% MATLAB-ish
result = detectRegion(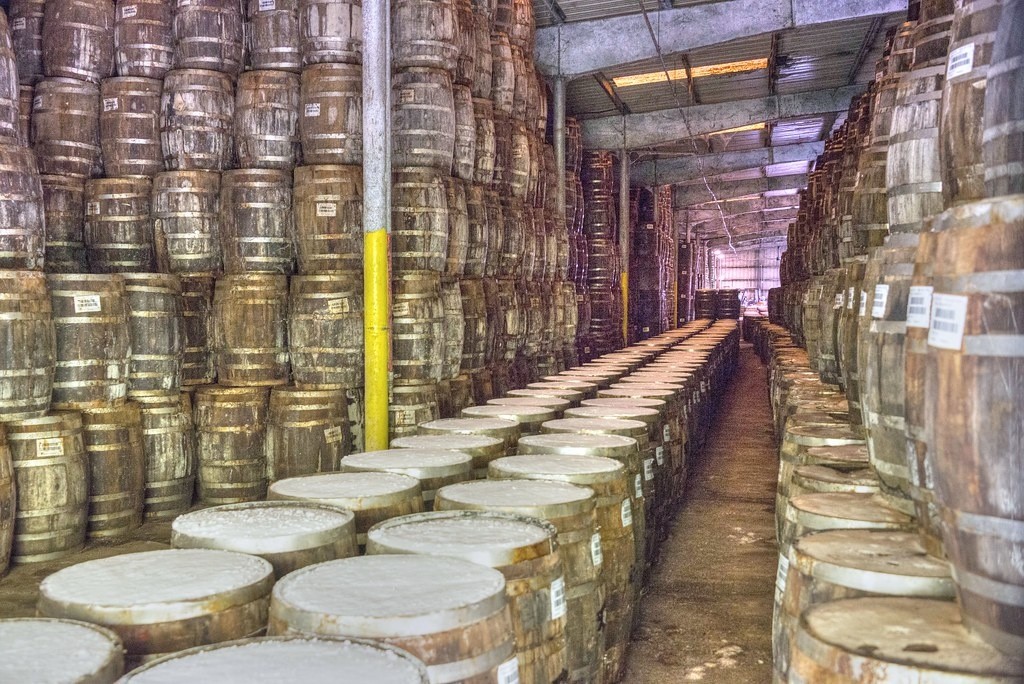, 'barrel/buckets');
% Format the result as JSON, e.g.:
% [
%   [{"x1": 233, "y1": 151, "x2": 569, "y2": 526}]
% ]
[
  {"x1": 1, "y1": 0, "x2": 740, "y2": 683},
  {"x1": 743, "y1": 0, "x2": 1024, "y2": 684}
]
